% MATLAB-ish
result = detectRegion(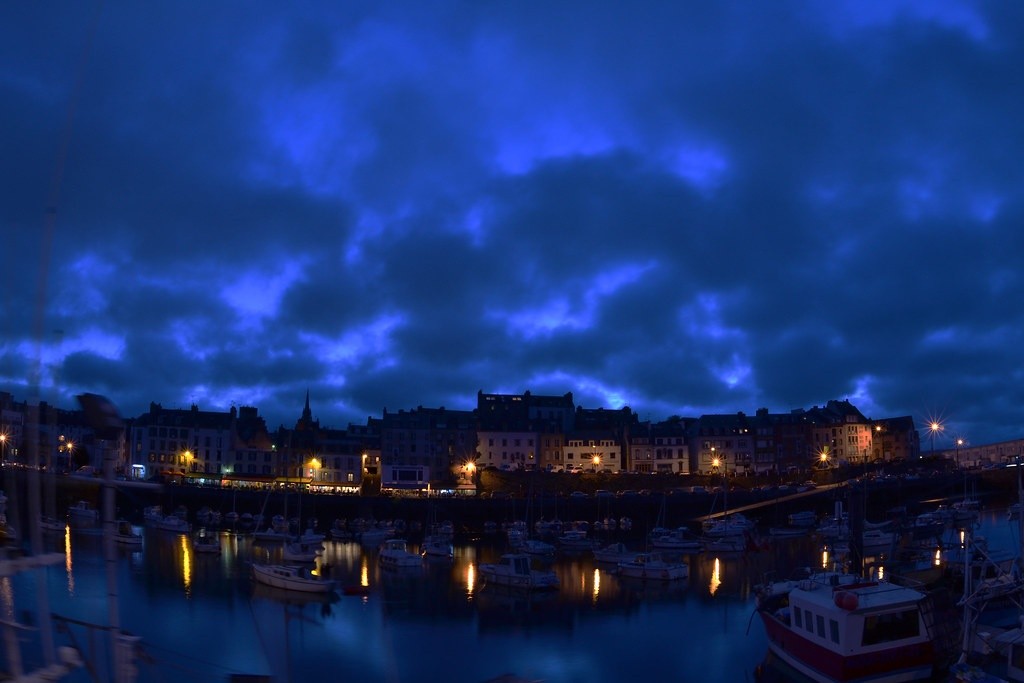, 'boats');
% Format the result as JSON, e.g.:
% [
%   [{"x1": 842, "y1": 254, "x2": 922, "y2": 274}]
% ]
[
  {"x1": 752, "y1": 552, "x2": 936, "y2": 683},
  {"x1": 0, "y1": 499, "x2": 1024, "y2": 683}
]
